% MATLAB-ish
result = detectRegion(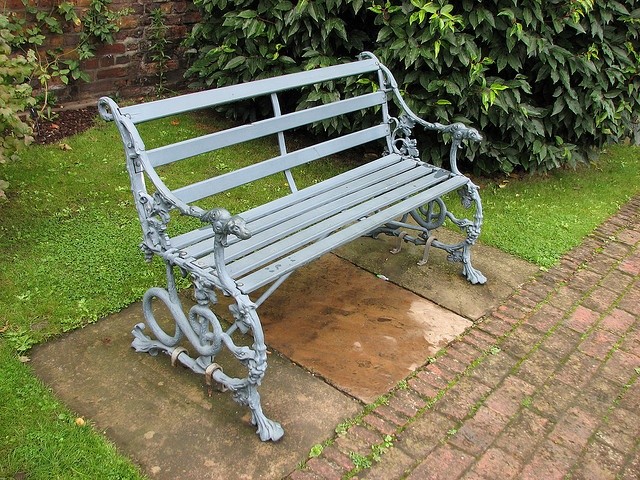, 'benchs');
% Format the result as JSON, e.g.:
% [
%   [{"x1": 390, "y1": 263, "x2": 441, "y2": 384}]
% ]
[{"x1": 98, "y1": 50, "x2": 487, "y2": 443}]
